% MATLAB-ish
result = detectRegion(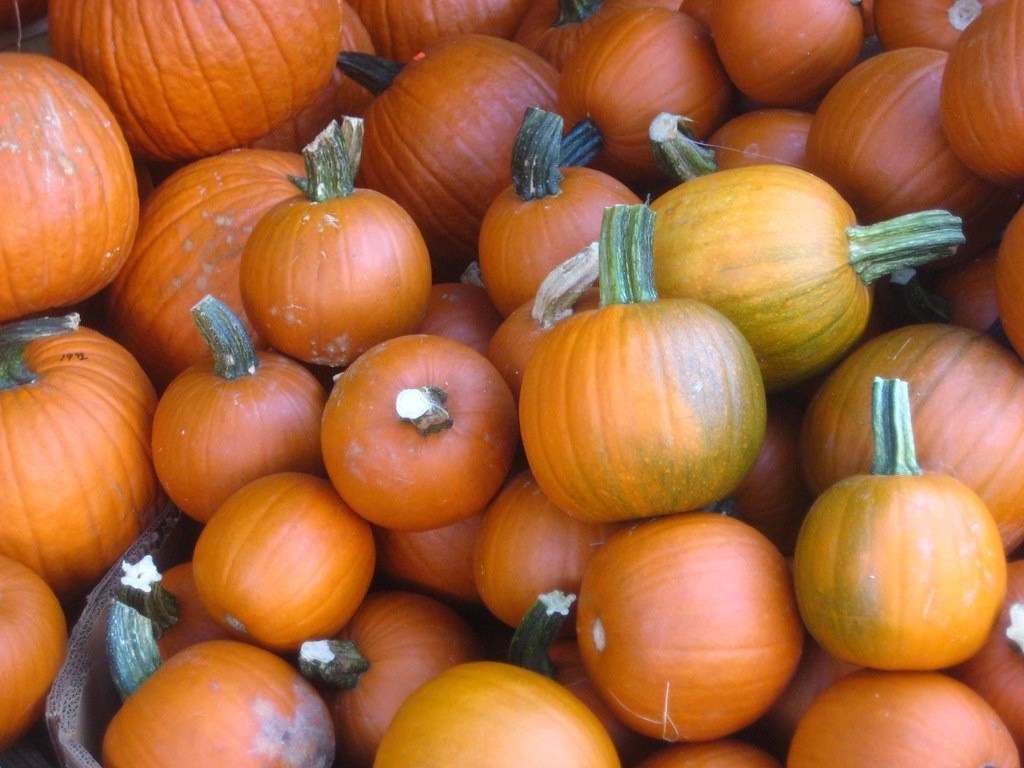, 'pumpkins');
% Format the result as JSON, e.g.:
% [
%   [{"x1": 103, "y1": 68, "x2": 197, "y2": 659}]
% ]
[{"x1": 0, "y1": 0, "x2": 1024, "y2": 768}]
